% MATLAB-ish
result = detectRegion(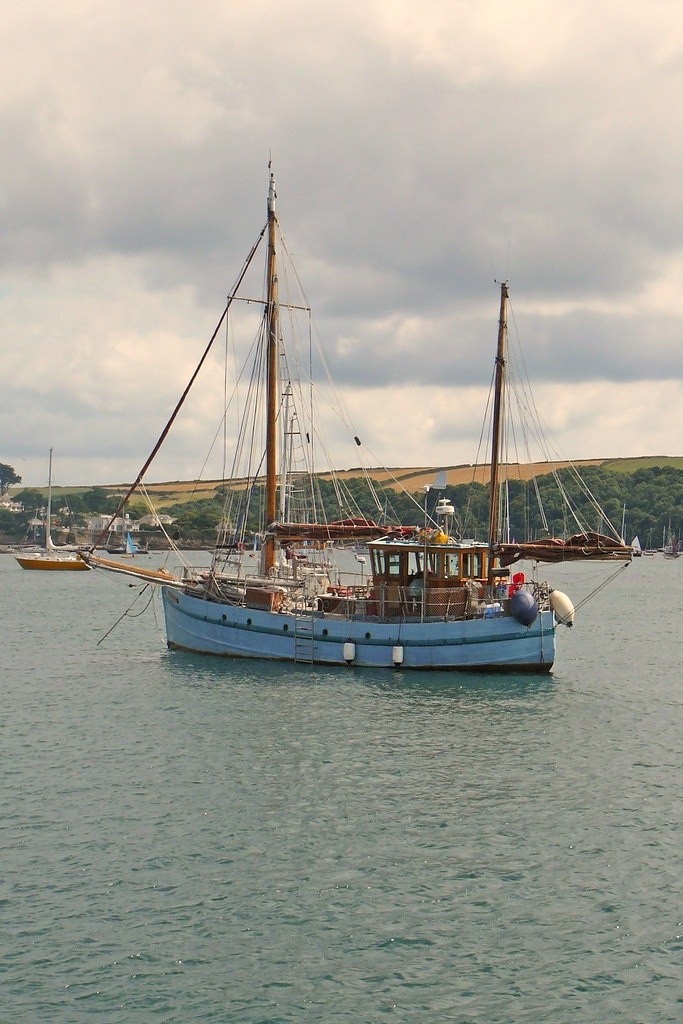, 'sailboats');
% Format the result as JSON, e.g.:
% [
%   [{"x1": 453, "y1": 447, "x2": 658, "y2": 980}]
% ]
[
  {"x1": 13, "y1": 449, "x2": 93, "y2": 571},
  {"x1": 0, "y1": 495, "x2": 683, "y2": 565},
  {"x1": 119, "y1": 530, "x2": 139, "y2": 558},
  {"x1": 65, "y1": 147, "x2": 641, "y2": 670}
]
